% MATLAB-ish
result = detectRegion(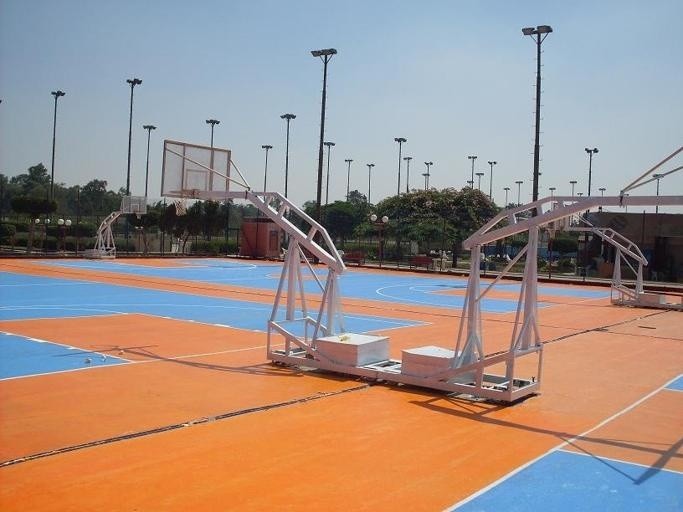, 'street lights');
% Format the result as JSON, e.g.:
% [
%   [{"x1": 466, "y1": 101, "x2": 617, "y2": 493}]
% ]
[
  {"x1": 262, "y1": 145, "x2": 272, "y2": 203},
  {"x1": 550, "y1": 181, "x2": 584, "y2": 225},
  {"x1": 404, "y1": 157, "x2": 413, "y2": 192},
  {"x1": 50, "y1": 91, "x2": 66, "y2": 201},
  {"x1": 206, "y1": 119, "x2": 220, "y2": 204},
  {"x1": 522, "y1": 25, "x2": 553, "y2": 229},
  {"x1": 599, "y1": 188, "x2": 606, "y2": 214},
  {"x1": 36, "y1": 219, "x2": 50, "y2": 248},
  {"x1": 422, "y1": 161, "x2": 433, "y2": 196},
  {"x1": 585, "y1": 147, "x2": 598, "y2": 213},
  {"x1": 653, "y1": 174, "x2": 664, "y2": 213},
  {"x1": 281, "y1": 114, "x2": 297, "y2": 200},
  {"x1": 395, "y1": 136, "x2": 407, "y2": 196},
  {"x1": 58, "y1": 219, "x2": 72, "y2": 252},
  {"x1": 344, "y1": 159, "x2": 352, "y2": 203},
  {"x1": 367, "y1": 163, "x2": 375, "y2": 204},
  {"x1": 127, "y1": 78, "x2": 143, "y2": 196},
  {"x1": 466, "y1": 155, "x2": 522, "y2": 210},
  {"x1": 371, "y1": 214, "x2": 390, "y2": 266},
  {"x1": 143, "y1": 124, "x2": 157, "y2": 196},
  {"x1": 311, "y1": 48, "x2": 338, "y2": 264}
]
